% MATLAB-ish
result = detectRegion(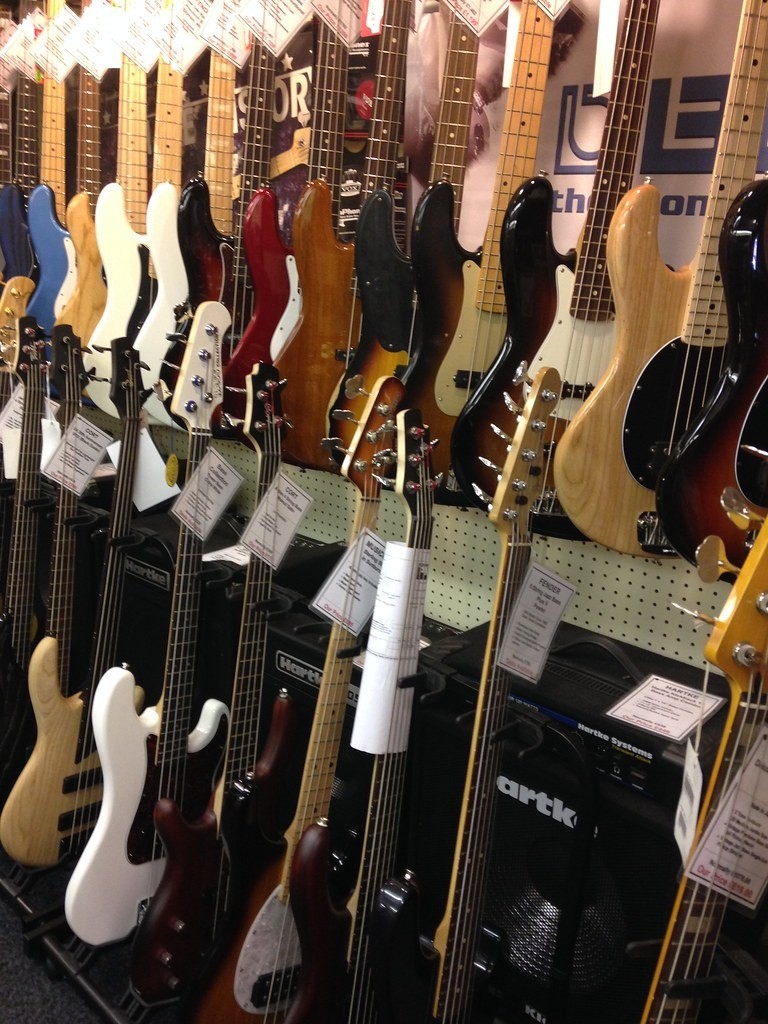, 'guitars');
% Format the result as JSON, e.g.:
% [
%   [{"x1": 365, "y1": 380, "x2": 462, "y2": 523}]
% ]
[
  {"x1": 1, "y1": 64, "x2": 100, "y2": 405},
  {"x1": 221, "y1": 12, "x2": 348, "y2": 453},
  {"x1": 1, "y1": 274, "x2": 147, "y2": 869},
  {"x1": 328, "y1": 0, "x2": 479, "y2": 475},
  {"x1": 129, "y1": 360, "x2": 291, "y2": 1004},
  {"x1": 64, "y1": 298, "x2": 234, "y2": 948},
  {"x1": 450, "y1": 1, "x2": 663, "y2": 544},
  {"x1": 184, "y1": 370, "x2": 768, "y2": 1024},
  {"x1": 156, "y1": 35, "x2": 277, "y2": 439},
  {"x1": 551, "y1": 0, "x2": 767, "y2": 561},
  {"x1": 50, "y1": 51, "x2": 149, "y2": 358},
  {"x1": 384, "y1": 0, "x2": 570, "y2": 507},
  {"x1": 81, "y1": 52, "x2": 185, "y2": 422},
  {"x1": 124, "y1": 47, "x2": 237, "y2": 435},
  {"x1": 267, "y1": 0, "x2": 413, "y2": 477},
  {"x1": 655, "y1": 178, "x2": 768, "y2": 586}
]
